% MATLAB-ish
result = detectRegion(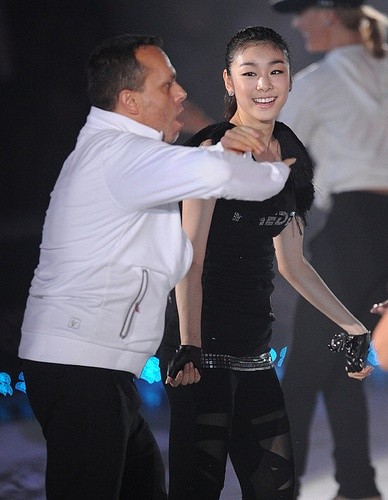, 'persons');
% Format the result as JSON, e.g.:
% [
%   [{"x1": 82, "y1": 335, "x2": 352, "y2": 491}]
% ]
[
  {"x1": 179, "y1": 0, "x2": 388, "y2": 500},
  {"x1": 157, "y1": 26, "x2": 373, "y2": 500},
  {"x1": 17, "y1": 34, "x2": 298, "y2": 500}
]
